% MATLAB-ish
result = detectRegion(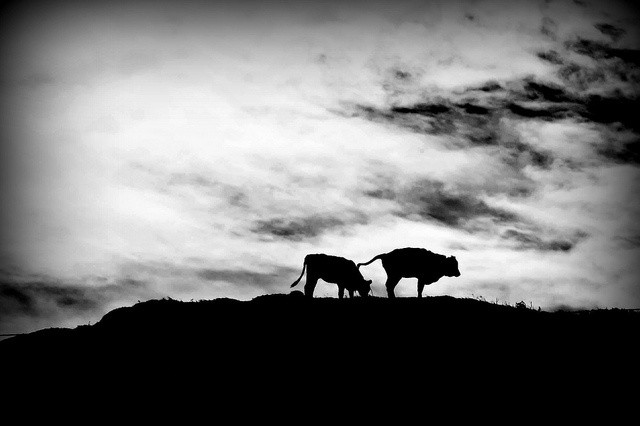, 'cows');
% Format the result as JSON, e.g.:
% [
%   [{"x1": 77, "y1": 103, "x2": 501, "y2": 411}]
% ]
[
  {"x1": 357, "y1": 248, "x2": 460, "y2": 298},
  {"x1": 290, "y1": 254, "x2": 372, "y2": 299}
]
